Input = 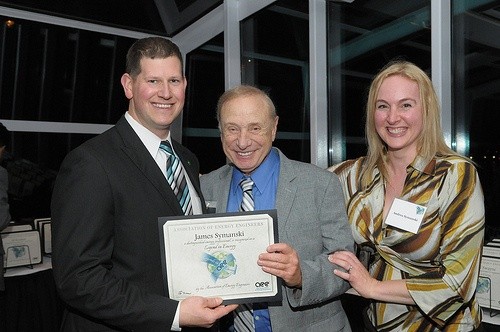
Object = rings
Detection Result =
[347,266,352,271]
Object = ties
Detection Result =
[233,178,255,332]
[159,140,194,216]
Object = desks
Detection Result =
[0,254,64,332]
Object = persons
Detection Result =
[51,38,239,332]
[199,85,354,332]
[0,123,9,295]
[326,60,485,332]
